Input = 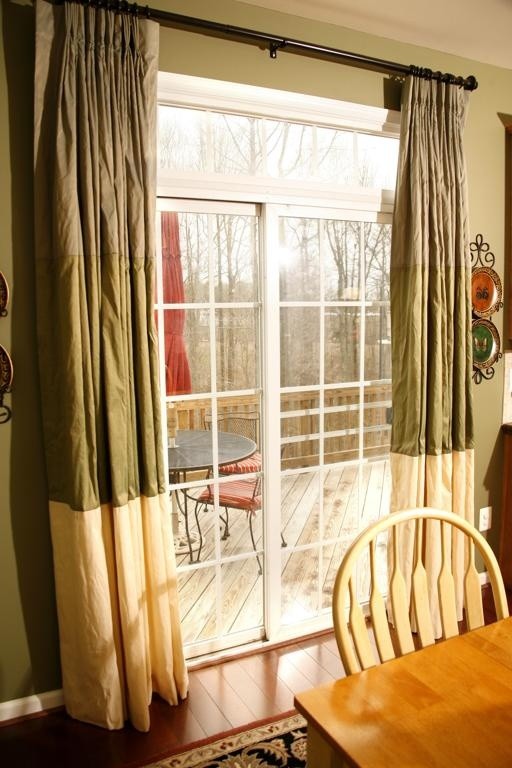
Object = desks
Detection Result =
[165,429,258,564]
[292,615,512,767]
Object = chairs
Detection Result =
[331,507,510,675]
[183,445,288,575]
[203,409,263,512]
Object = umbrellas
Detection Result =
[159,207,193,532]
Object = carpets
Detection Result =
[121,708,308,768]
